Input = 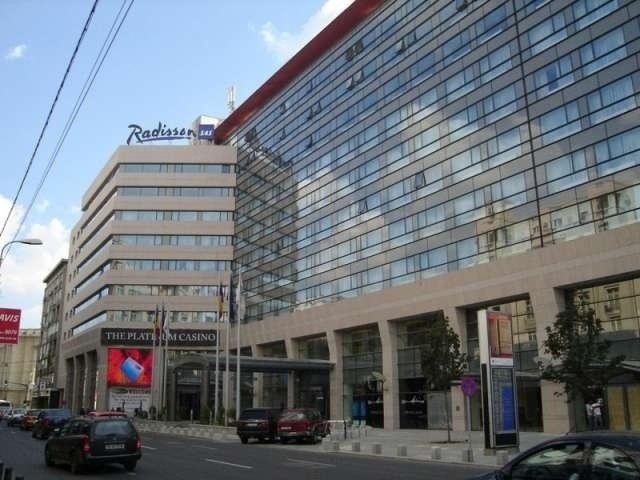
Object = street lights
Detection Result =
[4,380,29,409]
[0,237,44,267]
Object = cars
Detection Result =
[464,431,640,480]
[0,406,144,474]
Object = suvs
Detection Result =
[234,406,287,445]
[275,406,331,445]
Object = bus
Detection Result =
[0,400,13,417]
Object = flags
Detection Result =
[218,283,225,323]
[163,308,168,336]
[153,306,160,344]
[234,275,247,321]
